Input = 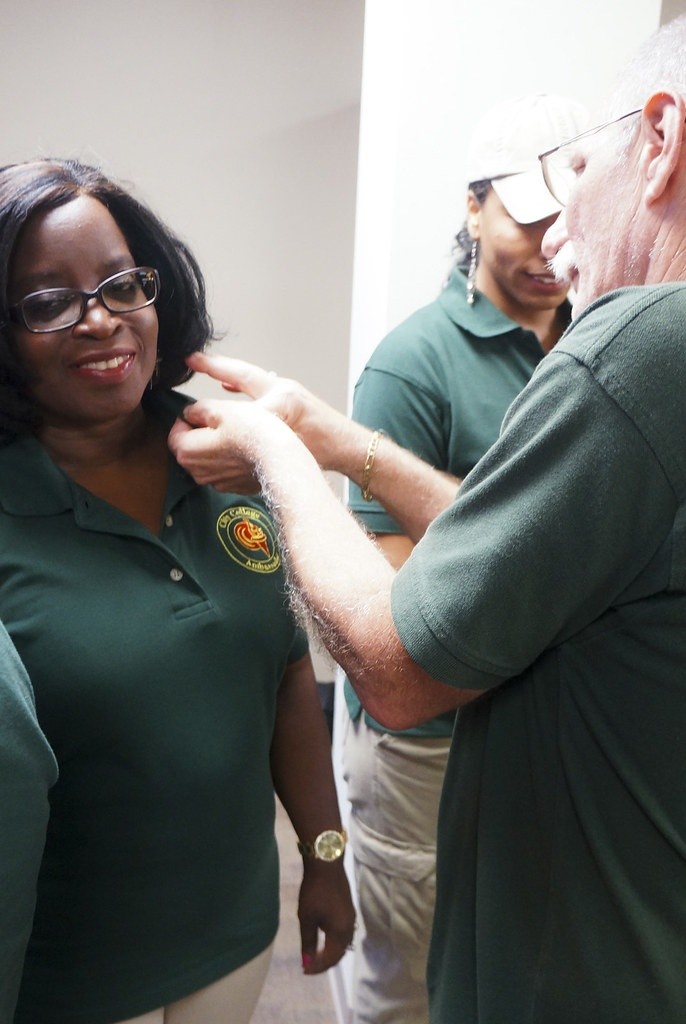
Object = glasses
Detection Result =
[16,267,159,333]
[542,107,642,208]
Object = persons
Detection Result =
[327,93,574,1024]
[0,157,363,1023]
[168,8,686,1024]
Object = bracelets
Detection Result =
[360,428,382,502]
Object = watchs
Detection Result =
[292,830,349,862]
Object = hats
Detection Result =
[469,143,564,224]
